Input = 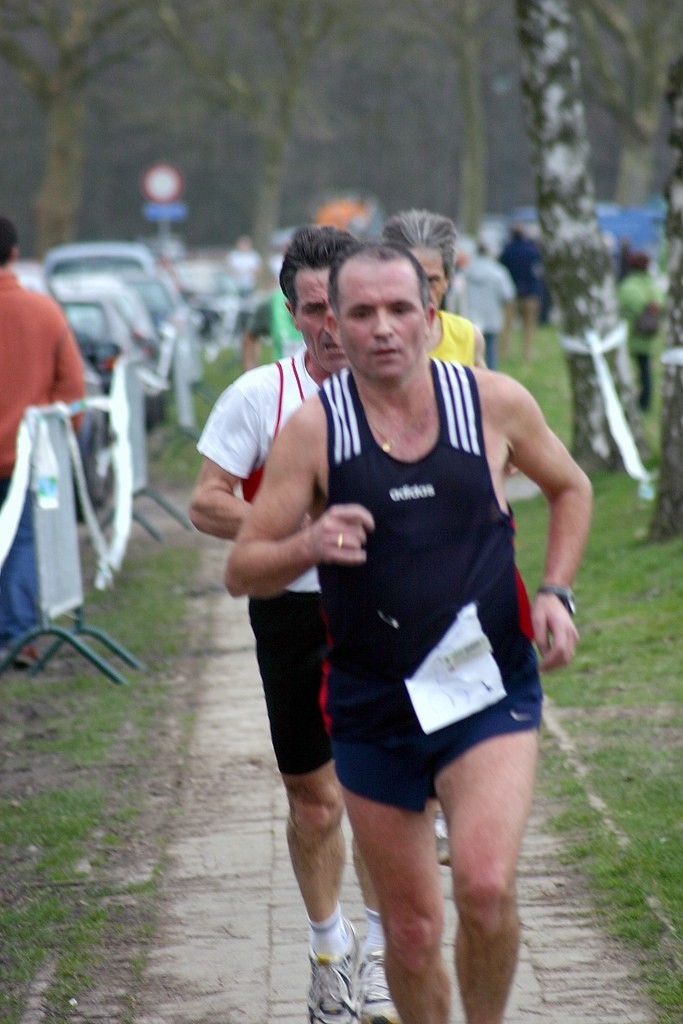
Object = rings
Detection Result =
[337,533,343,548]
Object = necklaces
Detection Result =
[363,400,418,452]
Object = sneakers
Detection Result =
[308,918,360,1024]
[357,949,400,1024]
[433,810,450,865]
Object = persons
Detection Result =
[500,224,547,357]
[222,239,592,1023]
[620,253,661,409]
[228,235,261,334]
[447,241,518,370]
[380,210,519,867]
[242,254,284,371]
[188,225,405,1024]
[0,218,84,667]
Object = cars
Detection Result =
[14,215,297,519]
[504,196,666,320]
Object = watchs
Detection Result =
[538,583,576,615]
